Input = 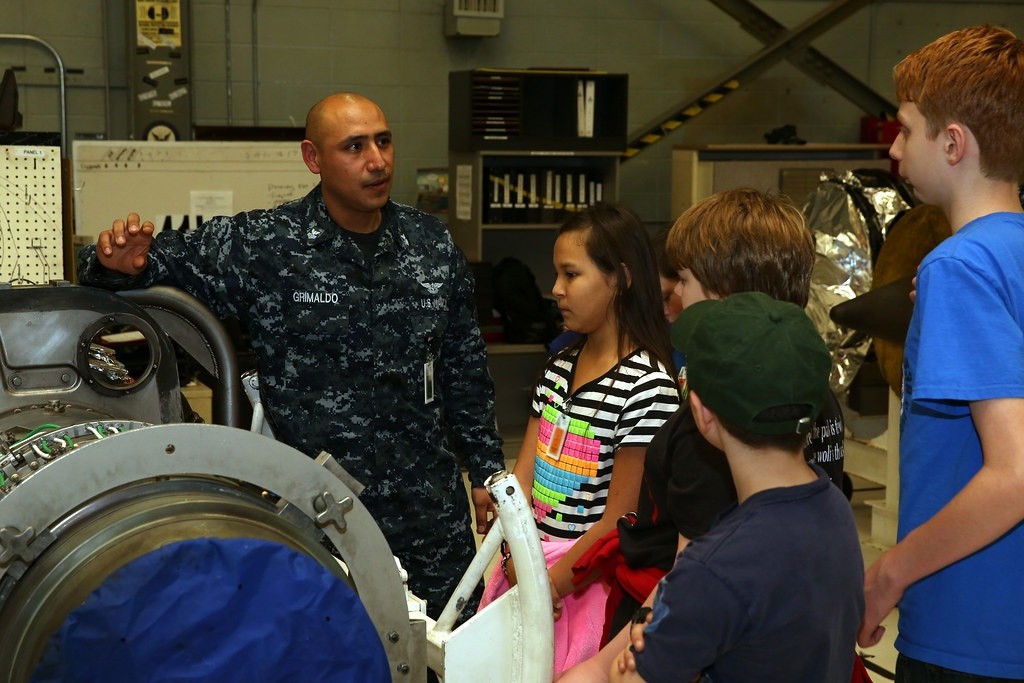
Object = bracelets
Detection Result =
[500,552,511,575]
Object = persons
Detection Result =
[607,290,865,683]
[475,200,679,679]
[77,91,505,683]
[553,186,845,683]
[857,24,1024,683]
